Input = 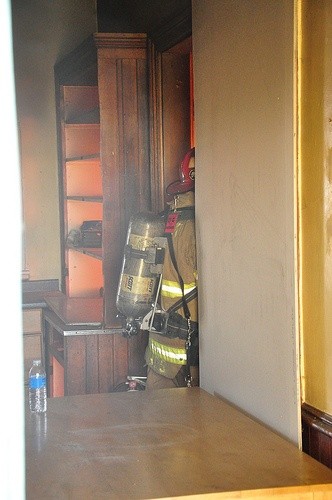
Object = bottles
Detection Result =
[28,359,47,413]
[115,210,166,337]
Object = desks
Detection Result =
[24,386,331,500]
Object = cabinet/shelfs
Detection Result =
[53,32,159,330]
[42,308,150,398]
[22,279,59,367]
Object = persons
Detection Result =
[145,146,201,388]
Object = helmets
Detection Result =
[166,146,195,196]
